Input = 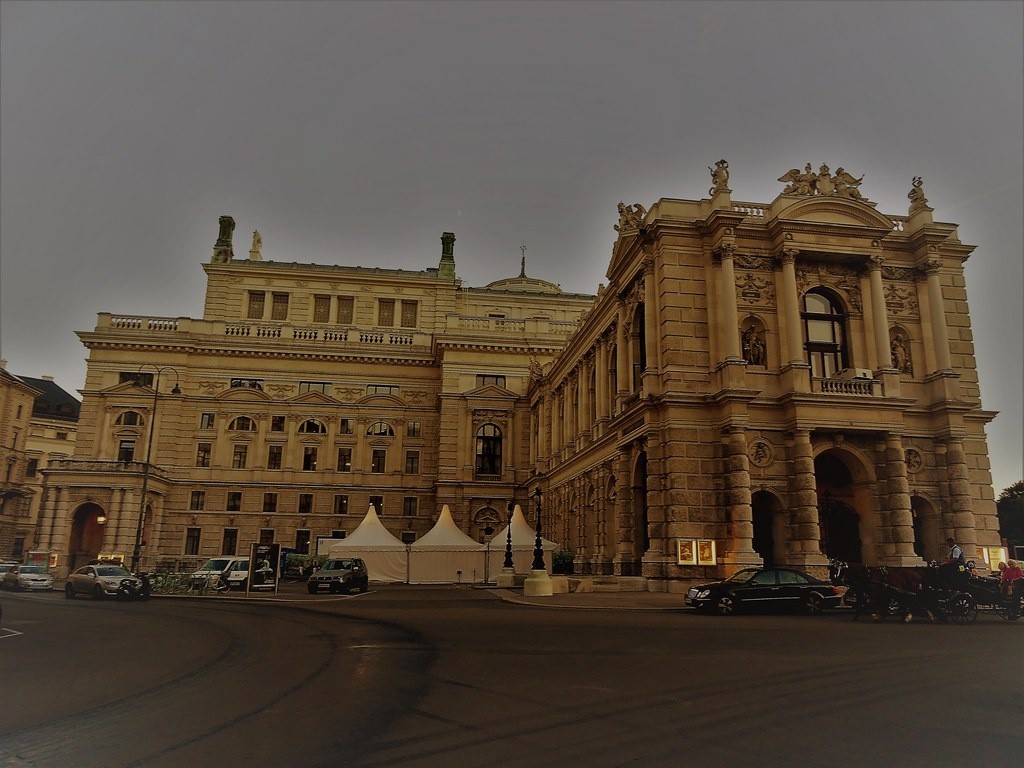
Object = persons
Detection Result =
[998,559,1023,600]
[617,202,643,232]
[908,176,928,214]
[890,333,908,373]
[780,162,817,195]
[743,324,764,365]
[708,159,729,194]
[831,167,865,198]
[251,229,262,251]
[940,538,964,568]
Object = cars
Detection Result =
[0,562,54,592]
[684,567,842,615]
[307,557,368,594]
[66,564,143,599]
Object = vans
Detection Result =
[191,557,250,589]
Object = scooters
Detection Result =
[117,570,151,600]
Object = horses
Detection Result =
[846,567,938,624]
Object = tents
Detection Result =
[328,504,557,585]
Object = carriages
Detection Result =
[827,563,1024,625]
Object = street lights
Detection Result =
[131,363,181,572]
[524,485,553,596]
[497,503,516,587]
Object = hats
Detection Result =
[944,538,955,542]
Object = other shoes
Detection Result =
[940,586,949,593]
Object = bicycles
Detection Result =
[148,564,231,595]
